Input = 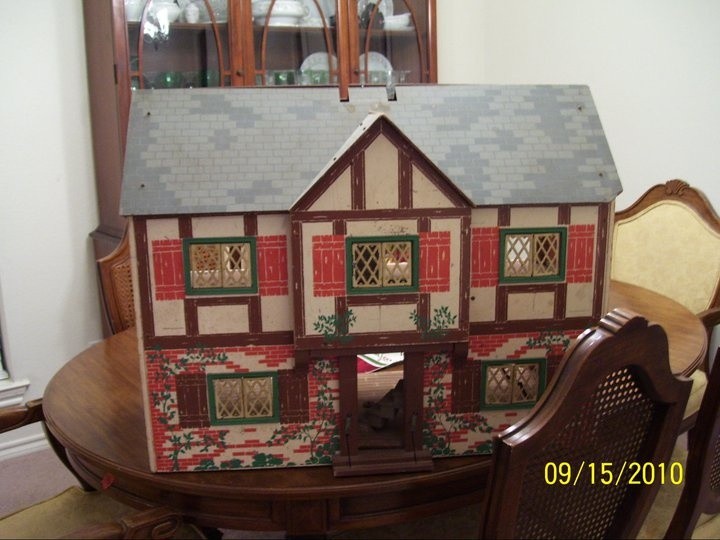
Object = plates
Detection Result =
[383,25,416,31]
[300,51,340,84]
[359,51,394,87]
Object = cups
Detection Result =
[131,67,413,90]
[184,3,199,22]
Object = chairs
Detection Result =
[667,346,720,540]
[93,220,135,339]
[606,179,719,316]
[1,398,209,540]
[362,309,693,540]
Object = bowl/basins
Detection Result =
[385,15,409,25]
[251,1,312,25]
[152,3,180,22]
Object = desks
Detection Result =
[43,281,707,540]
